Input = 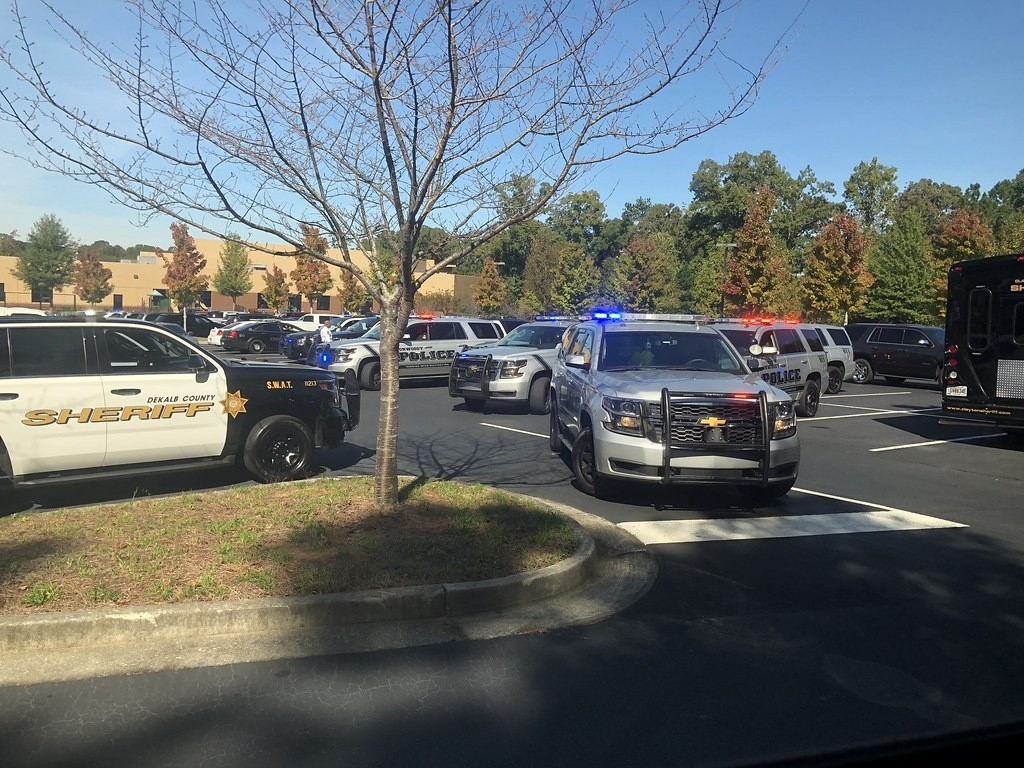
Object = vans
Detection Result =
[939,253,1024,436]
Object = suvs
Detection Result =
[304,313,506,390]
[0,311,364,516]
[447,314,585,415]
[279,315,381,360]
[702,317,830,419]
[545,310,800,504]
[842,323,945,386]
[807,323,856,394]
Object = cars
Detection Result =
[220,321,306,355]
[99,306,343,347]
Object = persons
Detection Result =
[233,316,239,322]
[321,321,332,343]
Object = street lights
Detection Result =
[715,243,739,318]
